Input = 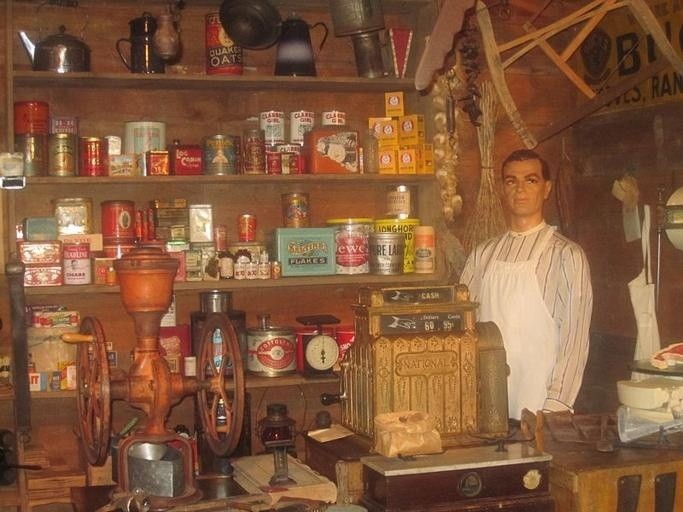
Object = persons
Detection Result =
[456,144,593,428]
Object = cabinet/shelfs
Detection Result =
[11,61,445,511]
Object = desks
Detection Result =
[513,412,682,512]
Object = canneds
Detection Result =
[200,290,231,316]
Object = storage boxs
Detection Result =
[304,126,360,175]
[264,227,336,277]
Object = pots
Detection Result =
[218,1,281,50]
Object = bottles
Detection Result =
[274,12,331,77]
[113,12,169,74]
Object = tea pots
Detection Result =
[17,25,94,72]
